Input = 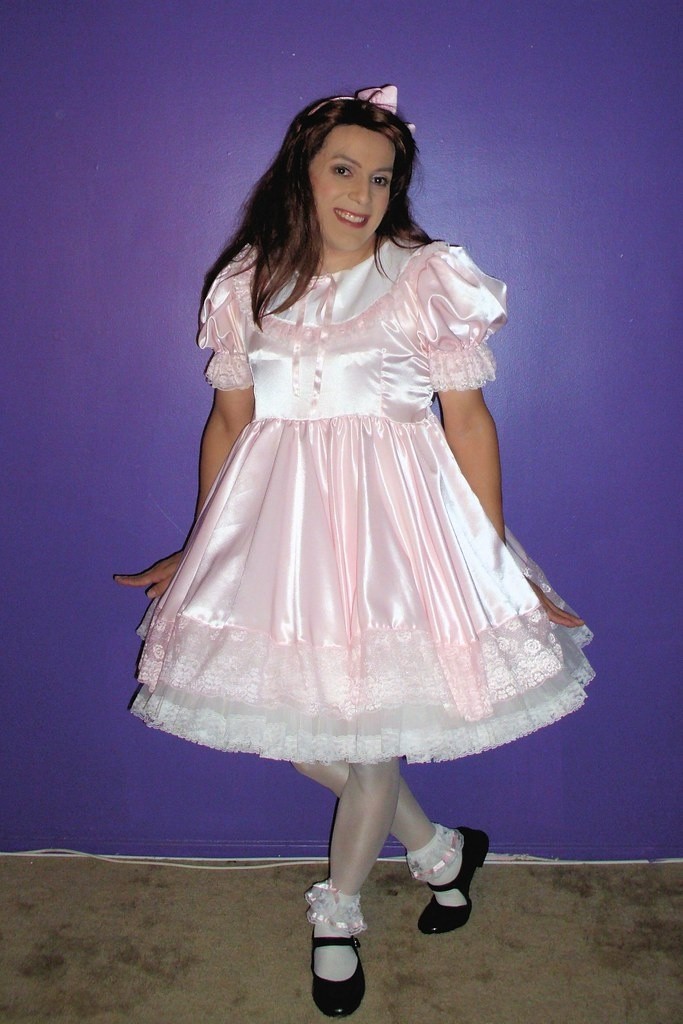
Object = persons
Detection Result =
[108,84,599,1019]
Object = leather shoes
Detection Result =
[310,920,365,1018]
[417,826,490,934]
